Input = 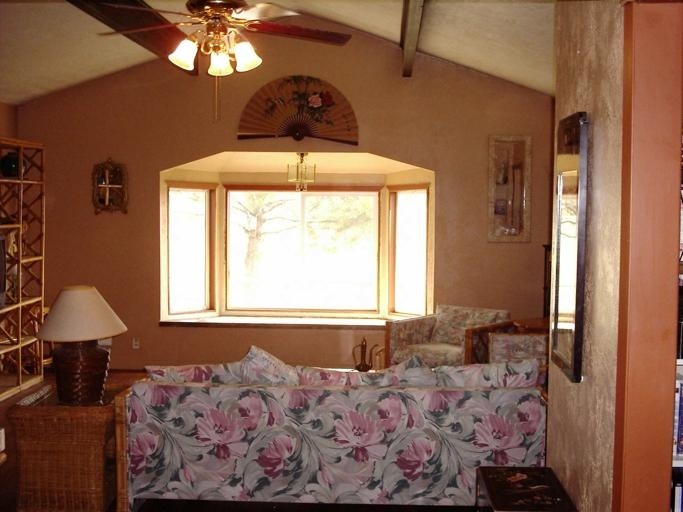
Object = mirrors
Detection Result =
[550,108,586,385]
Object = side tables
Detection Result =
[6,382,130,512]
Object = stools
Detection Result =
[474,465,579,511]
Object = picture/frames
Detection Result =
[92,156,127,215]
[487,135,533,244]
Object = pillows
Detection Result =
[432,358,537,385]
[294,366,435,385]
[242,346,301,385]
[143,360,240,382]
[380,356,422,372]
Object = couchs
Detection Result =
[126,381,546,508]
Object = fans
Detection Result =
[96,1,352,47]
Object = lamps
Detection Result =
[34,285,128,406]
[167,21,263,77]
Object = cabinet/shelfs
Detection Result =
[1,137,44,402]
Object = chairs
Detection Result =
[385,302,549,365]
